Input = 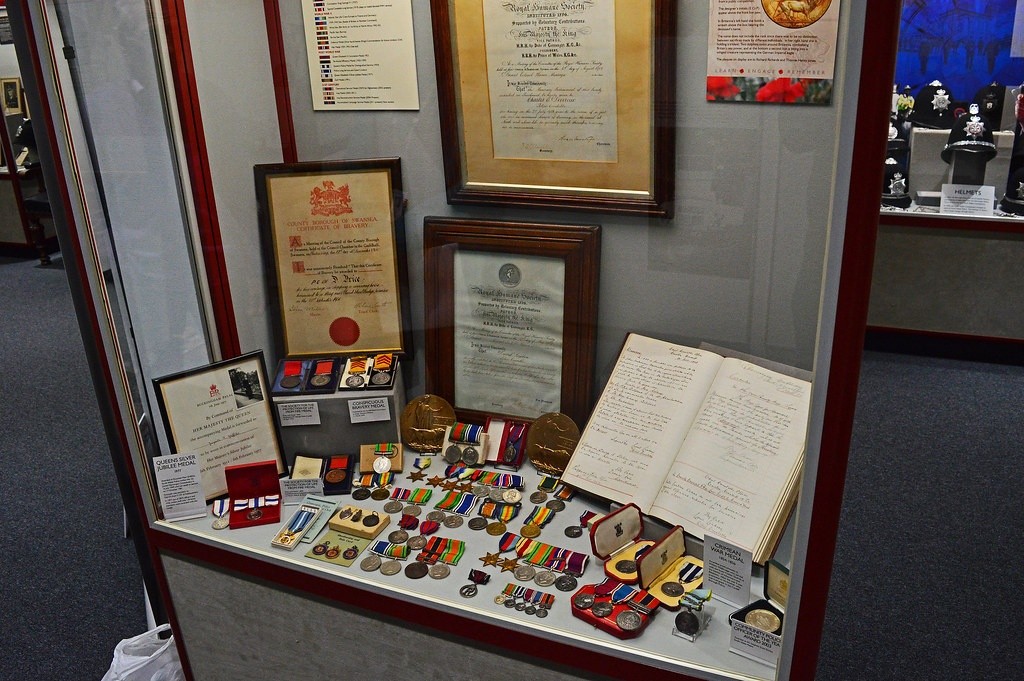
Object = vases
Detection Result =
[896,108,911,123]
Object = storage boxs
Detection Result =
[270,354,409,462]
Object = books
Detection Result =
[561,333,813,568]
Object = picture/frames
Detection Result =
[0,77,23,113]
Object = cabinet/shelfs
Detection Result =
[0,0,1024,681]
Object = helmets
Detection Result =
[939,101,1000,162]
[906,78,958,128]
[974,81,1014,135]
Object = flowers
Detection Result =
[897,93,915,110]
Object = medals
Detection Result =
[212,353,780,644]
